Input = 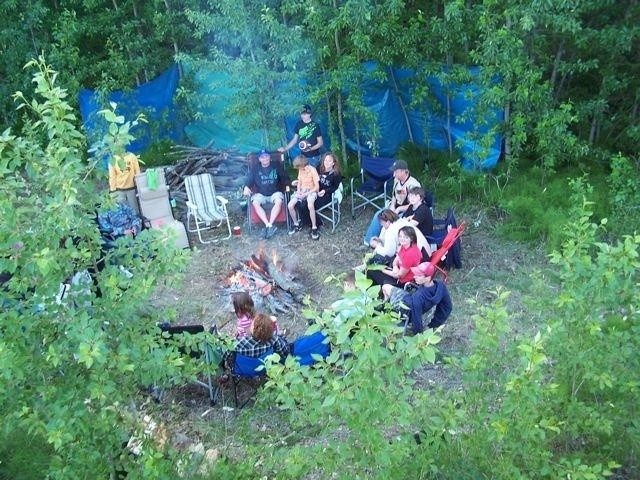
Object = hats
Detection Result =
[387,159,408,171]
[411,261,435,278]
[257,148,269,157]
[302,105,311,114]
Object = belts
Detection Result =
[116,187,137,192]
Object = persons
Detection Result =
[229,291,257,337]
[352,161,422,254]
[366,225,423,287]
[369,207,432,260]
[106,140,142,217]
[221,314,290,372]
[400,186,434,244]
[380,261,454,336]
[242,149,290,238]
[276,103,324,169]
[286,155,322,240]
[295,151,342,236]
[97,200,154,270]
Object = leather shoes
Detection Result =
[216,372,230,385]
[244,377,261,389]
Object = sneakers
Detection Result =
[288,220,324,240]
[120,269,133,278]
[265,225,277,238]
[257,226,267,241]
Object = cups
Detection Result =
[234,226,241,235]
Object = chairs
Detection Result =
[156,324,331,411]
[402,189,465,336]
[133,150,397,259]
